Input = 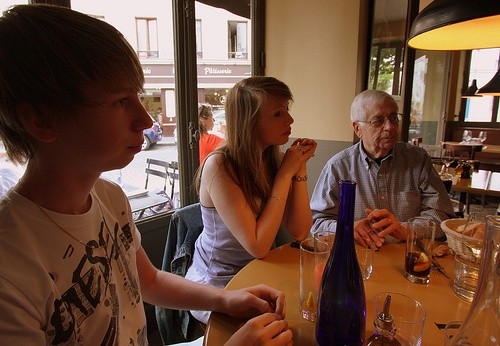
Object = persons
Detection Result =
[0,4,294,346]
[184,74,317,323]
[156,106,162,124]
[310,89,454,252]
[197,101,227,166]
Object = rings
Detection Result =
[301,148,305,154]
[388,217,392,223]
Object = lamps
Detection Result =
[407,0,500,51]
[461,79,482,98]
[474,68,500,96]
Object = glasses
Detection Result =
[357,113,404,128]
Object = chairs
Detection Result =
[443,144,482,161]
[136,157,179,219]
[155,203,206,345]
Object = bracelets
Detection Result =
[291,174,308,182]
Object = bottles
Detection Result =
[364,294,401,346]
[454,161,471,179]
[447,215,500,345]
[316,180,364,346]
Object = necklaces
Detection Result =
[38,191,110,249]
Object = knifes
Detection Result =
[417,239,451,280]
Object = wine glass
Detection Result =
[441,149,455,173]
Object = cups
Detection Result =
[445,320,497,346]
[365,291,425,346]
[438,173,453,195]
[299,230,377,322]
[441,218,487,303]
[463,129,487,146]
[404,217,436,282]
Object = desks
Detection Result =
[202,234,500,346]
[443,141,500,162]
[434,164,500,196]
[129,192,169,219]
